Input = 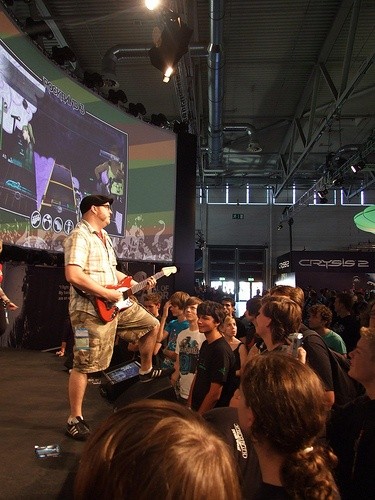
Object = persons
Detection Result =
[245,297,267,352]
[236,351,341,500]
[21,123,35,170]
[75,400,241,500]
[326,327,375,500]
[217,315,247,390]
[57,283,236,371]
[95,147,124,234]
[229,296,307,406]
[309,304,347,360]
[188,301,235,413]
[0,238,18,337]
[254,276,375,359]
[64,194,167,439]
[156,292,190,370]
[127,293,163,360]
[171,297,207,404]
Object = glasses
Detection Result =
[94,205,113,211]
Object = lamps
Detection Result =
[351,161,365,173]
[148,21,194,73]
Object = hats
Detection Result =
[80,194,114,214]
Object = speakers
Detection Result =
[113,375,179,417]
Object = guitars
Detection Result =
[95,265,177,324]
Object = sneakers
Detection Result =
[64,416,92,441]
[138,367,175,384]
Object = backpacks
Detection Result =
[303,330,365,410]
